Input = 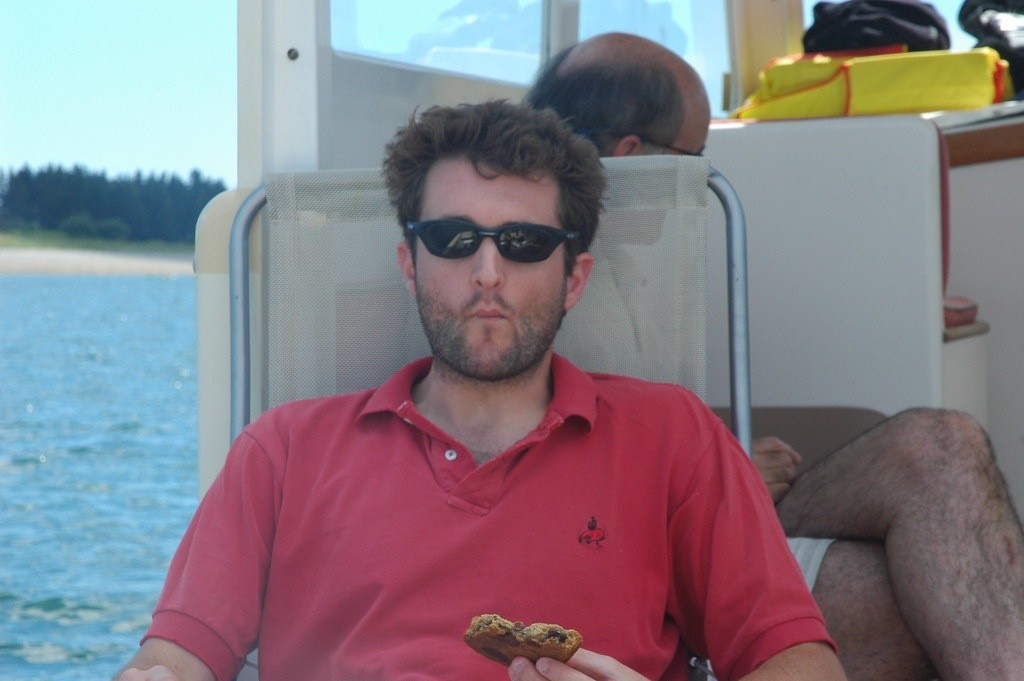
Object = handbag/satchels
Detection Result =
[800,1,950,52]
[731,41,1008,121]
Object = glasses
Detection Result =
[405,219,581,264]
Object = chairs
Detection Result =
[229,153,755,462]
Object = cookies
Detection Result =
[463,614,584,666]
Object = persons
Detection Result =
[104,102,845,681]
[519,0,1024,679]
